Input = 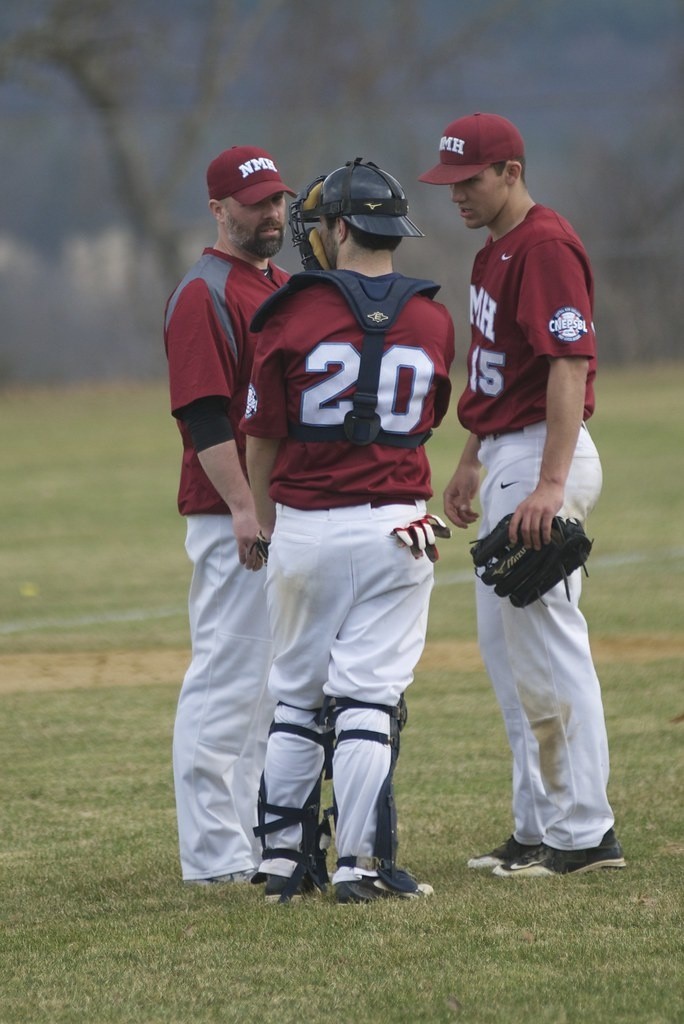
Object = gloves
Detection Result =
[392,514,452,564]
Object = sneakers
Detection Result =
[194,866,258,890]
[254,874,317,905]
[468,824,629,880]
[336,865,433,901]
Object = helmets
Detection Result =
[322,166,422,244]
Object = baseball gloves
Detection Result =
[470,513,594,611]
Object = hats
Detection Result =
[419,112,524,183]
[206,146,297,208]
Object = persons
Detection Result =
[161,146,323,881]
[412,112,627,875]
[230,160,459,907]
